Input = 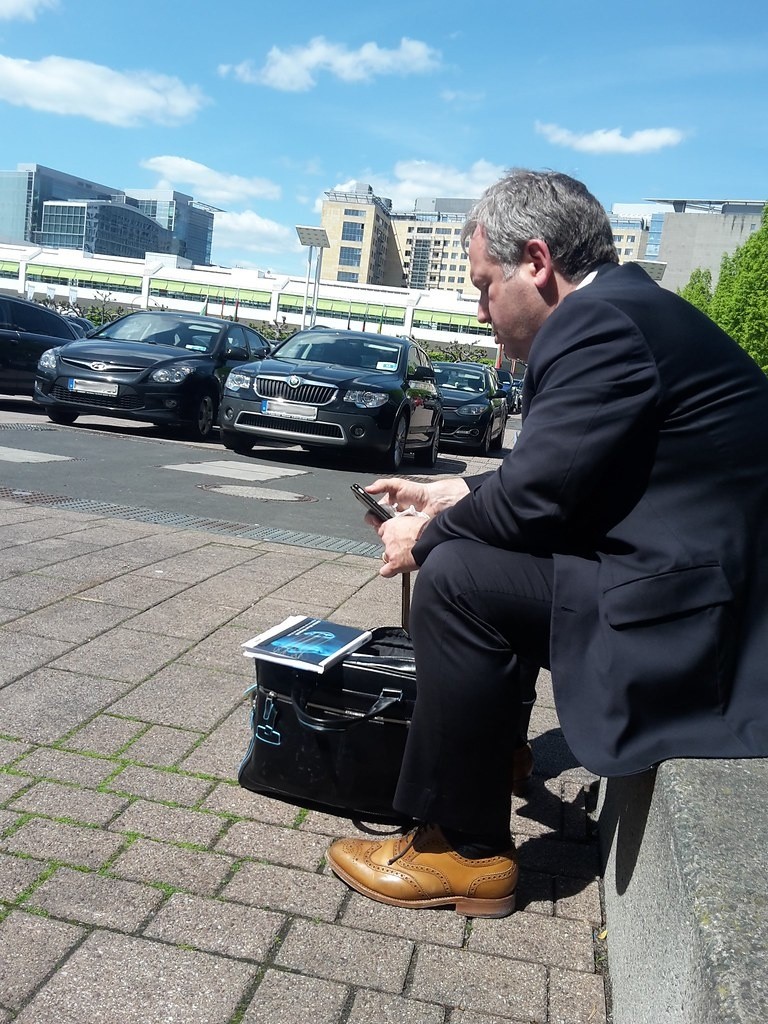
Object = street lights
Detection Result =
[296,225,331,327]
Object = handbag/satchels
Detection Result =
[237,626,423,820]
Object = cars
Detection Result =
[215,323,445,471]
[0,294,83,412]
[61,315,97,339]
[32,310,272,444]
[429,358,523,456]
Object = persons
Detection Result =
[326,167,767,920]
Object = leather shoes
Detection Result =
[324,821,519,920]
[512,744,534,794]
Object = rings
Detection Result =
[382,552,388,563]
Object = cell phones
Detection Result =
[351,483,393,521]
